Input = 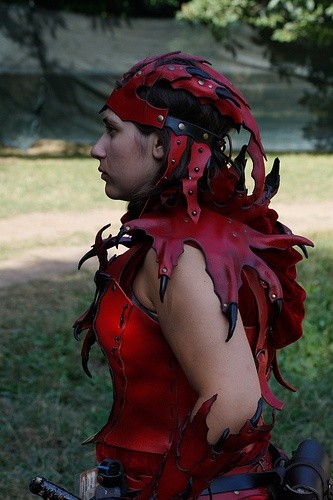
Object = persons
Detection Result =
[70,50,315,500]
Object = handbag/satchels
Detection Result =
[278,440,332,500]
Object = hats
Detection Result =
[99,51,281,224]
